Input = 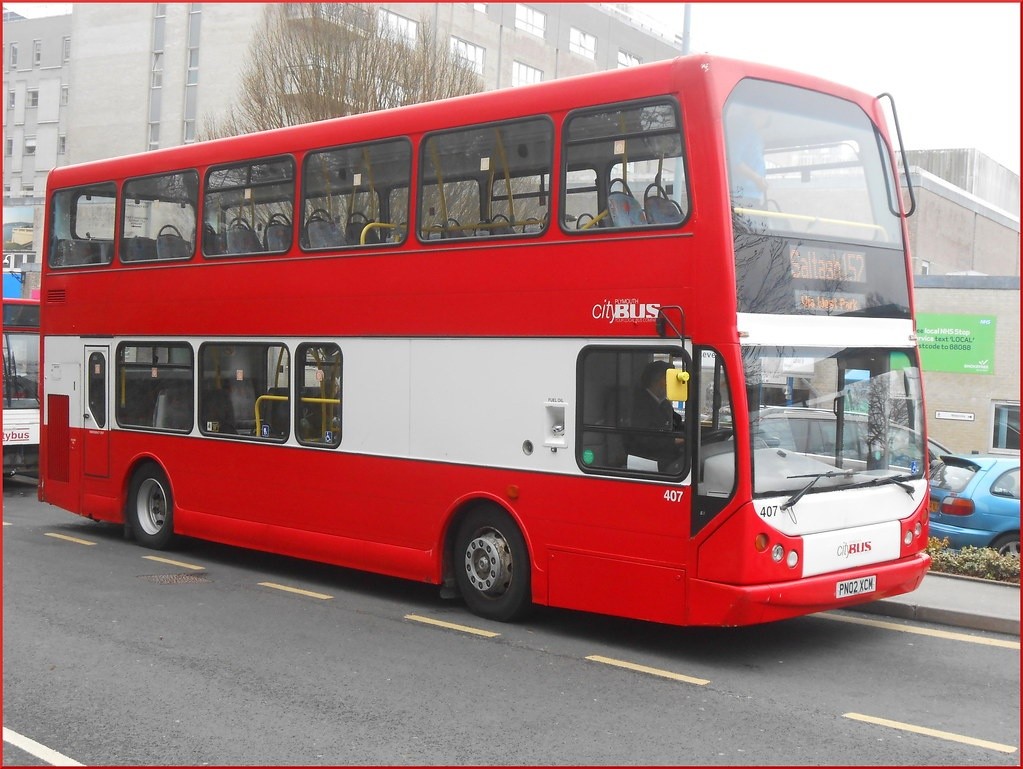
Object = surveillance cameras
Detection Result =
[3,260,9,264]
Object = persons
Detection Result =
[614,361,703,471]
[309,378,340,445]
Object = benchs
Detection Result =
[154,383,196,431]
[224,379,263,429]
[55,234,157,266]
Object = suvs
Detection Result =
[704,402,953,474]
[928,451,1021,559]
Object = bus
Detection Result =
[2,297,40,475]
[37,56,933,626]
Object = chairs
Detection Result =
[263,212,293,253]
[576,212,597,229]
[190,223,220,257]
[604,387,634,466]
[523,216,543,234]
[644,183,680,223]
[224,218,262,254]
[426,218,466,241]
[346,211,379,247]
[606,178,647,226]
[474,221,490,234]
[305,209,346,250]
[489,214,516,235]
[157,225,187,259]
